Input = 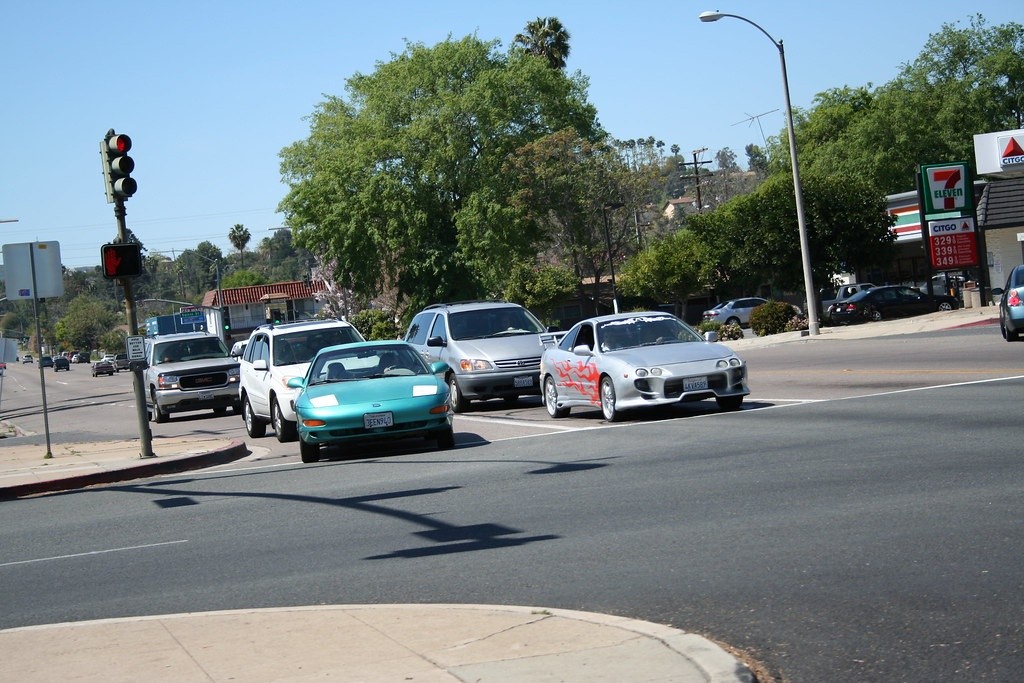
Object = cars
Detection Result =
[703,297,801,329]
[991,265,1024,341]
[287,340,454,463]
[23,354,33,364]
[539,312,750,422]
[803,275,965,328]
[51,351,90,363]
[37,356,69,372]
[91,361,113,377]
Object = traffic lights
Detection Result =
[104,133,138,198]
[222,306,231,340]
[271,308,282,324]
[101,242,143,279]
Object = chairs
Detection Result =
[328,361,346,380]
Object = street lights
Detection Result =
[699,10,820,335]
[152,249,222,310]
[602,203,624,314]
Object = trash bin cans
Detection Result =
[962,288,972,309]
[970,289,981,308]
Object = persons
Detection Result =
[277,334,346,362]
[597,325,672,352]
[159,342,217,363]
[378,353,403,376]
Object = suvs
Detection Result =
[231,317,380,443]
[143,331,240,424]
[101,353,130,372]
[402,299,558,413]
